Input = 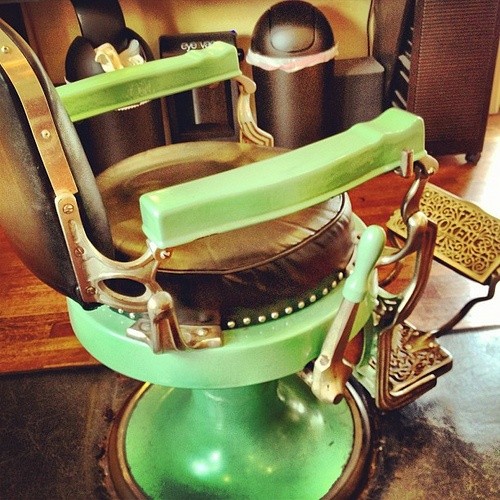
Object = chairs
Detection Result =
[0,21,500,500]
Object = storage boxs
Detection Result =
[333,56,385,132]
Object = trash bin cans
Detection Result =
[158,28,245,141]
[245,0,338,152]
[62,0,168,177]
